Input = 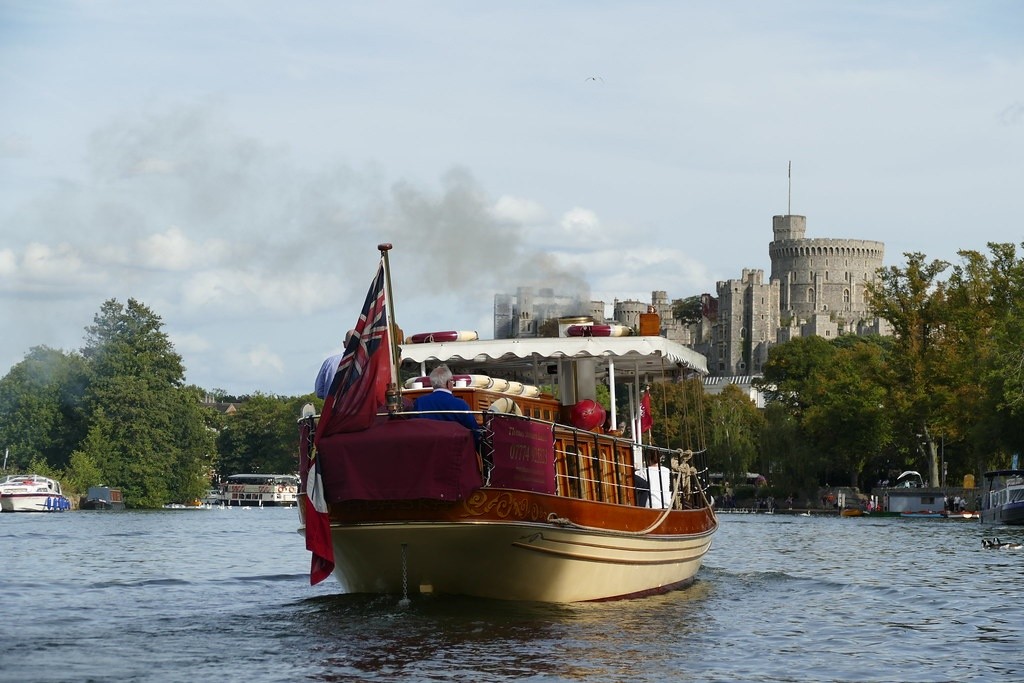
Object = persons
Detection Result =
[414,364,479,430]
[822,480,982,512]
[635,444,672,509]
[616,414,632,439]
[314,329,356,401]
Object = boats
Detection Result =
[841,508,863,517]
[0,473,70,512]
[217,473,298,507]
[202,490,221,503]
[981,537,1021,549]
[183,497,201,506]
[288,238,722,606]
[977,468,1024,526]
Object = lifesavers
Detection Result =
[404,374,540,388]
[405,330,478,344]
[558,322,635,337]
[481,397,522,477]
[289,486,295,493]
[278,486,284,492]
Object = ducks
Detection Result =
[981,537,1024,551]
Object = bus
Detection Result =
[709,473,768,496]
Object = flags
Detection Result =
[298,265,390,585]
[635,392,653,434]
